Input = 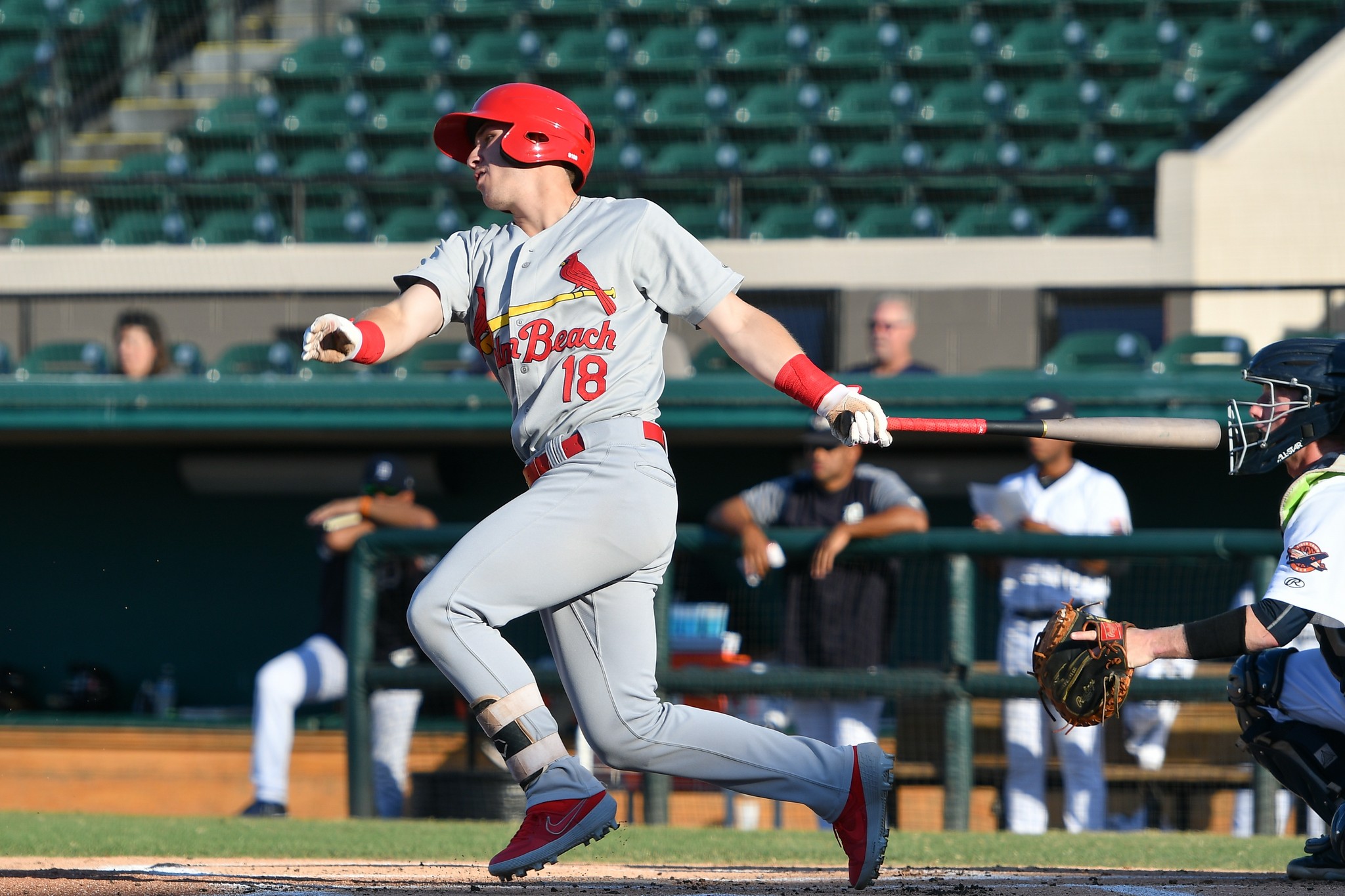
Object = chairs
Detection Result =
[1039,331,1152,373]
[1151,335,1250,373]
[690,336,748,374]
[17,338,484,375]
[0,0,1345,242]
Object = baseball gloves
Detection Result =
[1032,608,1137,727]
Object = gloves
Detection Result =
[301,313,362,361]
[817,384,892,448]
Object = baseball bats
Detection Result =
[840,410,1221,451]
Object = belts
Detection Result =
[1012,603,1085,621]
[522,421,665,489]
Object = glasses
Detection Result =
[868,321,893,329]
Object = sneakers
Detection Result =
[488,790,618,881]
[832,743,895,890]
[1287,853,1345,881]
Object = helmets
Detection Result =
[433,82,595,193]
[1226,337,1345,475]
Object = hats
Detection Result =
[364,460,418,494]
[1023,393,1074,420]
[799,413,841,446]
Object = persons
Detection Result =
[1069,336,1345,883]
[972,391,1132,834]
[1121,656,1203,769]
[714,408,930,828]
[113,308,171,378]
[239,454,443,817]
[301,82,895,890]
[841,298,940,376]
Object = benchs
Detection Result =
[874,663,1261,835]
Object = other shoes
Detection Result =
[243,801,285,818]
